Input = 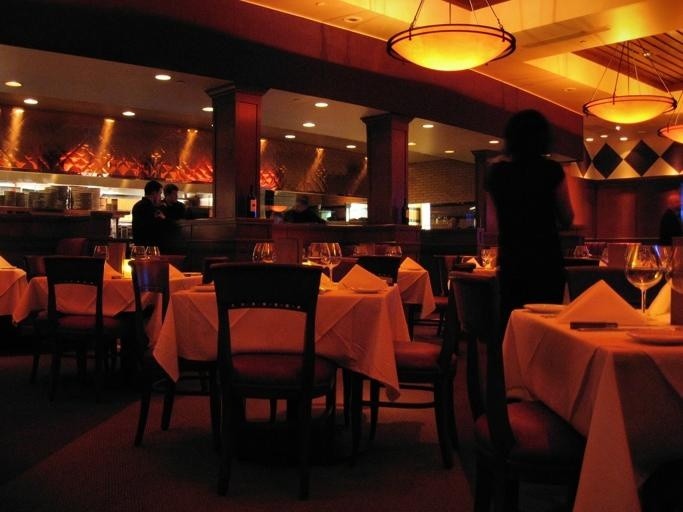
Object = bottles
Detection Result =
[106,197,119,211]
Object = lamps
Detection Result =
[582,39,678,125]
[386,0,517,72]
[656,90,683,145]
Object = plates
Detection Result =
[193,285,216,292]
[626,327,683,347]
[352,288,378,293]
[0,185,107,213]
[523,303,566,312]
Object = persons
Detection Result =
[658,189,682,247]
[160,184,185,245]
[479,109,574,340]
[284,197,326,223]
[131,180,165,248]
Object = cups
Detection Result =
[131,246,161,259]
[94,246,110,262]
[251,243,277,264]
[666,246,683,295]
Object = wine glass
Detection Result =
[481,249,496,271]
[309,243,343,283]
[624,242,665,322]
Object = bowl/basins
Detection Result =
[455,263,476,272]
